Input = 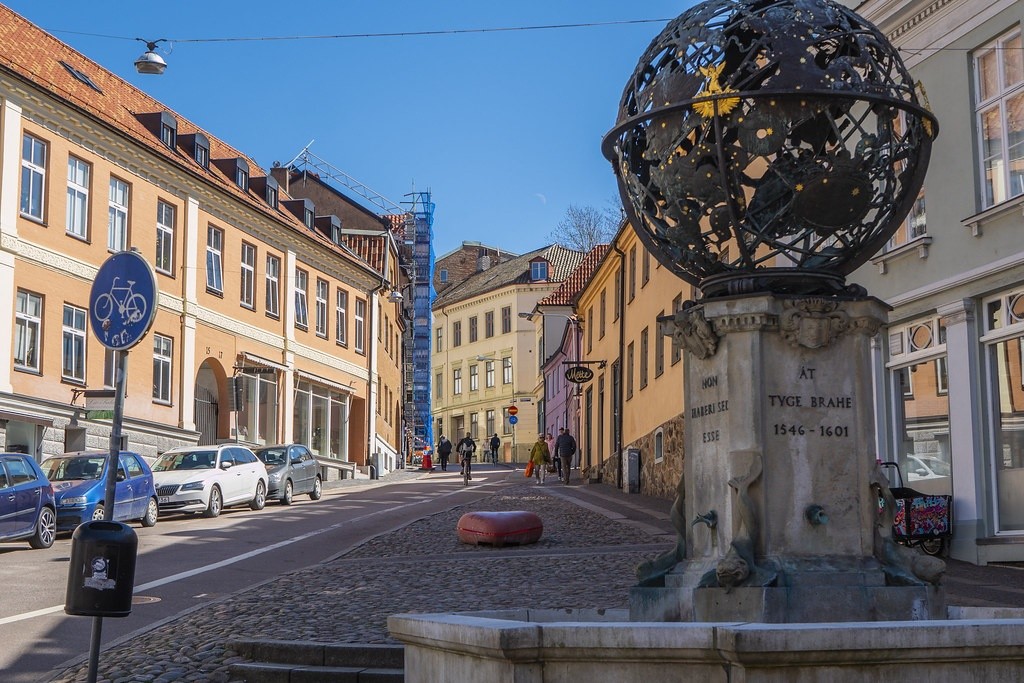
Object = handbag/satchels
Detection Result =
[525,461,534,477]
[552,456,561,469]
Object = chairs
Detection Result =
[63,460,99,479]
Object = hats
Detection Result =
[559,427,564,431]
[484,438,488,440]
[539,433,544,437]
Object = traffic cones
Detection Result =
[419,445,436,471]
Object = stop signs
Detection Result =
[508,406,518,415]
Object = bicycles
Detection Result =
[485,451,490,462]
[458,451,474,486]
[490,447,499,467]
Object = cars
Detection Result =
[907,453,951,482]
[39,450,159,531]
[254,443,322,505]
[149,443,268,517]
[0,452,57,548]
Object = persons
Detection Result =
[490,433,500,463]
[482,439,490,463]
[437,435,452,471]
[198,454,210,466]
[555,427,576,484]
[176,456,190,467]
[97,464,123,482]
[545,434,555,472]
[456,431,476,480]
[530,433,550,484]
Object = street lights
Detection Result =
[477,355,519,462]
[517,311,580,468]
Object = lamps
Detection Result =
[385,285,404,303]
[130,37,174,75]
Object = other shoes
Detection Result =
[562,478,564,481]
[536,480,540,484]
[468,475,472,480]
[460,470,464,475]
[558,477,561,480]
[566,481,569,485]
[541,482,545,484]
